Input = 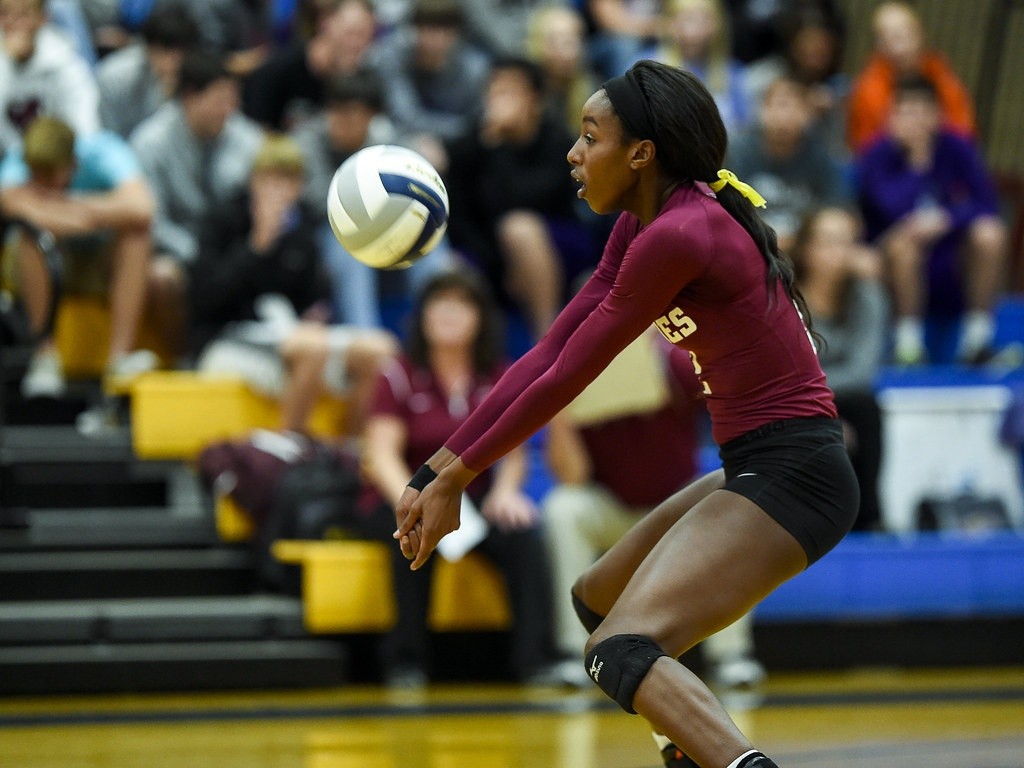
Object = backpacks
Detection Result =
[197,424,363,540]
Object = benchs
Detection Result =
[46,293,1024,625]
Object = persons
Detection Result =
[0,0,1024,696]
[395,58,860,768]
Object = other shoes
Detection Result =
[736,752,781,768]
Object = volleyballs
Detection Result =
[325,143,450,271]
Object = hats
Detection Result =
[251,132,312,175]
[173,56,231,95]
[22,117,75,164]
